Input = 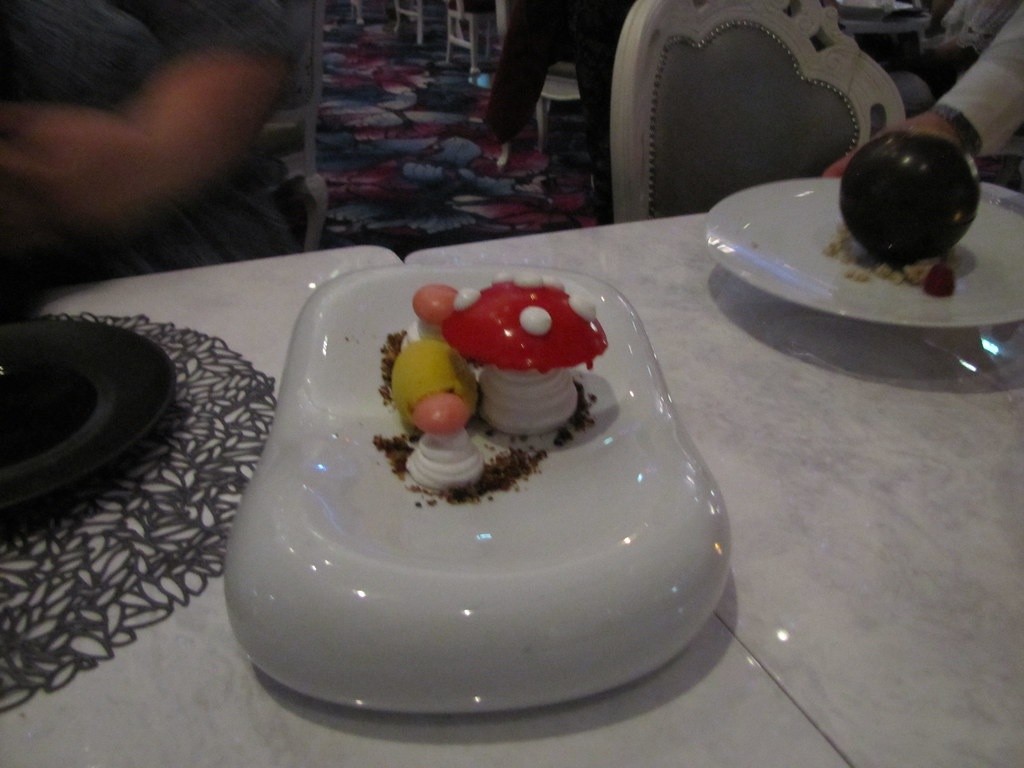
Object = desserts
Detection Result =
[377,270,611,507]
[834,127,983,300]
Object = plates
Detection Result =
[703,176,1024,328]
[222,260,733,718]
[1,320,177,511]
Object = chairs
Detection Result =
[608,1,908,226]
[259,1,335,252]
[348,0,587,154]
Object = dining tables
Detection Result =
[0,178,1021,765]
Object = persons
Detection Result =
[0,0,312,308]
[823,0,1024,179]
[483,0,631,225]
